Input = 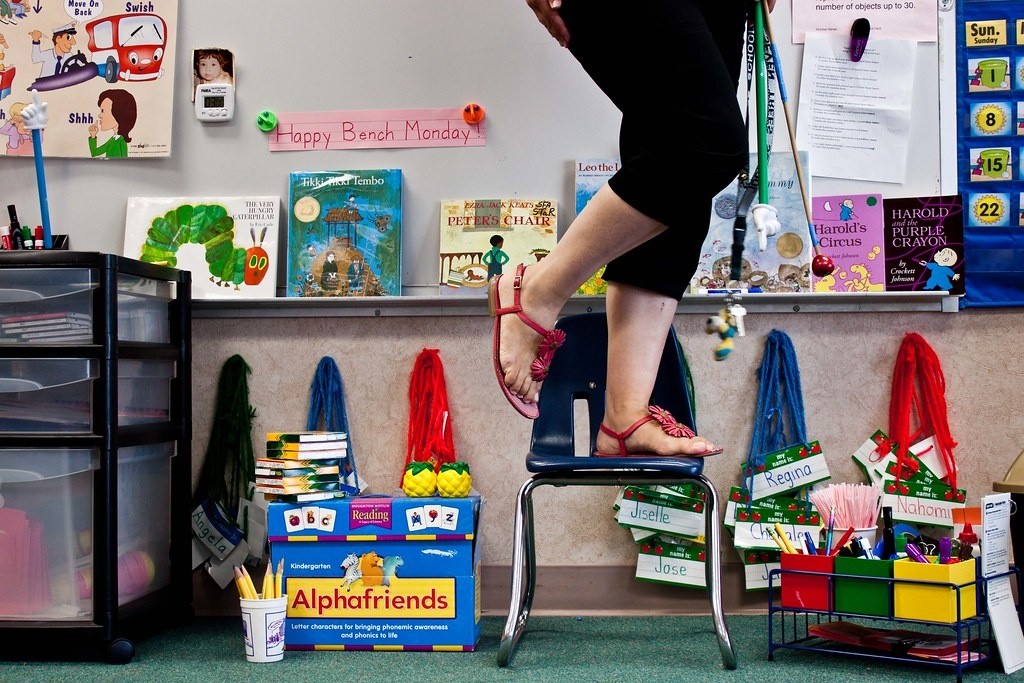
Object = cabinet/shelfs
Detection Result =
[769,563,1024,683]
[0,250,198,662]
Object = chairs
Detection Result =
[495,311,738,671]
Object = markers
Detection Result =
[906,537,952,564]
[7,203,44,250]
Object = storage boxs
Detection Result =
[891,556,979,624]
[834,556,910,617]
[267,493,478,541]
[780,548,841,610]
[270,541,484,651]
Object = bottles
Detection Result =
[958,521,980,558]
[896,534,907,557]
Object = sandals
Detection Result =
[489,263,565,419]
[594,405,724,458]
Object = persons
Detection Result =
[193,49,233,96]
[487,1,777,459]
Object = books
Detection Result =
[880,196,967,296]
[252,431,351,505]
[437,197,559,296]
[689,150,814,295]
[0,312,92,347]
[573,156,624,216]
[286,167,403,299]
[811,194,886,294]
[121,196,280,301]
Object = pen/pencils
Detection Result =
[232,558,285,598]
[766,507,854,555]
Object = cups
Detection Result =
[824,526,878,553]
[240,594,288,662]
